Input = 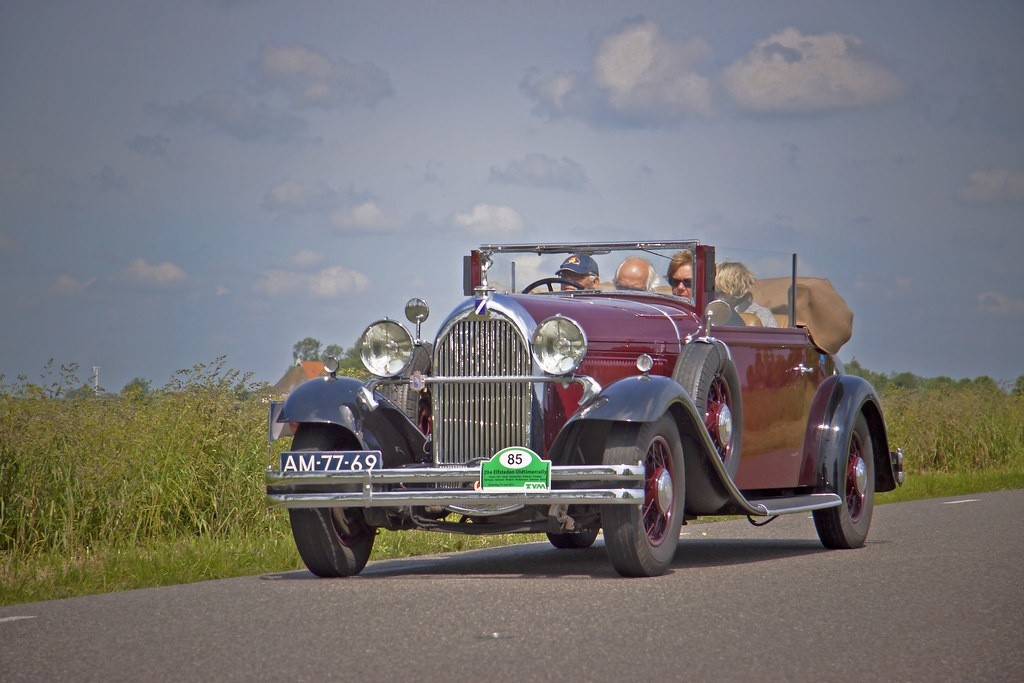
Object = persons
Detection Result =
[714,256,777,328]
[555,255,600,292]
[664,251,694,298]
[612,257,661,292]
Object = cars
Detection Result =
[264,236,908,579]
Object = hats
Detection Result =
[556,254,599,276]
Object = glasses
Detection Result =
[669,277,692,288]
[616,283,647,291]
[559,272,595,280]
